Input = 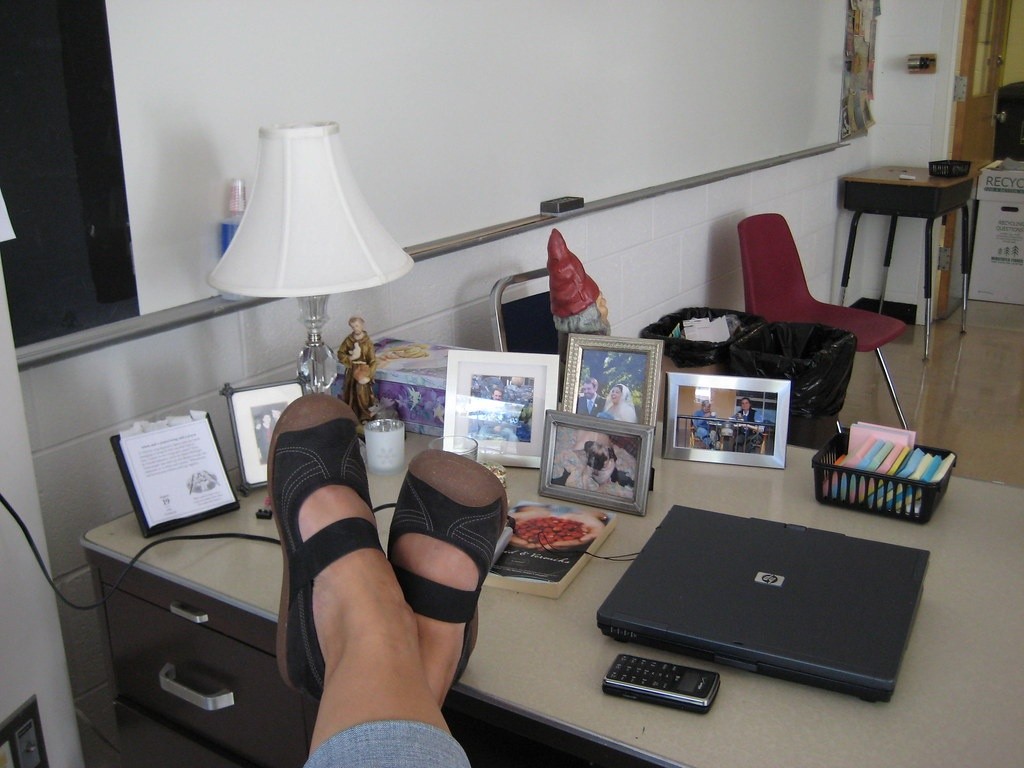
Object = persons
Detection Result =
[576,376,637,423]
[266,390,509,768]
[469,372,532,442]
[338,318,378,421]
[255,408,282,461]
[692,397,765,451]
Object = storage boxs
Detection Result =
[332,332,480,439]
[970,158,1024,304]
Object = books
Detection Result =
[483,500,618,599]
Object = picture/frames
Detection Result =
[538,410,656,516]
[221,374,311,496]
[662,371,792,470]
[443,347,560,467]
[562,332,664,429]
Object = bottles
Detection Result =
[222,180,250,300]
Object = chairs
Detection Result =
[738,213,909,435]
[487,268,559,356]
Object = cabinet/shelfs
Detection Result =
[81,445,1024,768]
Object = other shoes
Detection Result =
[266,392,387,696]
[386,447,508,685]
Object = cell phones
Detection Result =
[602,654,721,715]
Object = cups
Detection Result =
[429,436,478,462]
[364,419,405,476]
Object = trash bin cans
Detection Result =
[639,305,767,426]
[728,322,858,452]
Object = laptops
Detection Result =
[597,505,930,704]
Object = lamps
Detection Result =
[209,122,413,393]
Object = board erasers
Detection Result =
[539,195,585,216]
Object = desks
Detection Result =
[842,164,981,358]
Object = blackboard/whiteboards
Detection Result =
[0,0,851,371]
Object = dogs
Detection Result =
[561,441,617,483]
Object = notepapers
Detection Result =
[824,421,955,512]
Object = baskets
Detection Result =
[929,160,971,178]
[812,433,956,523]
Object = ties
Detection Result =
[588,400,593,414]
